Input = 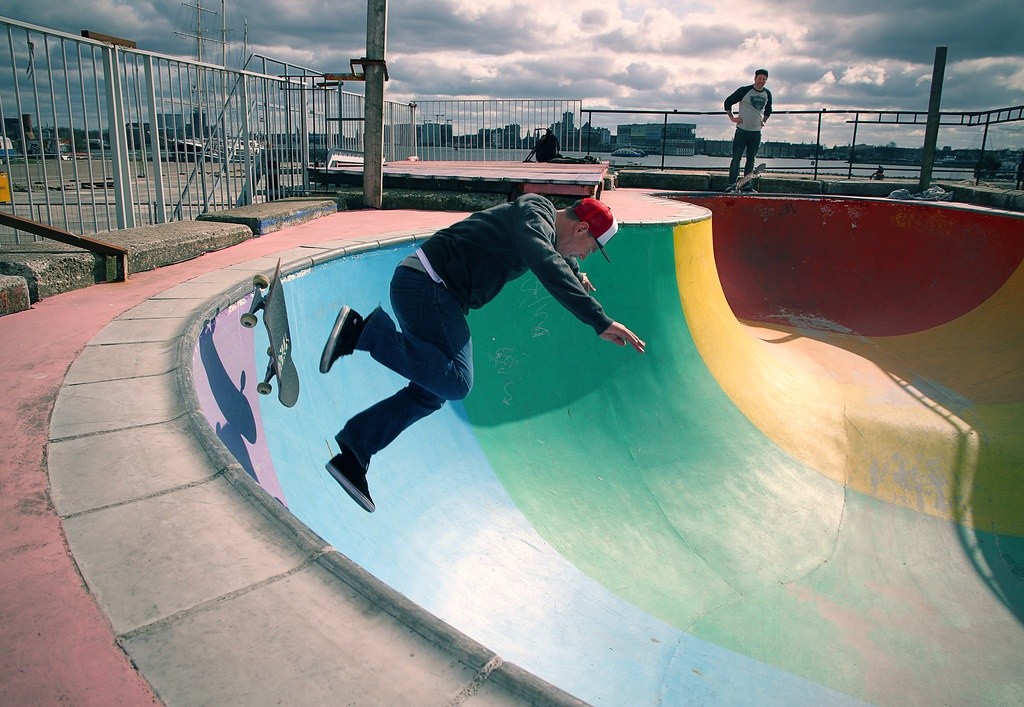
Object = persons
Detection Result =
[320,193,648,513]
[724,68,772,194]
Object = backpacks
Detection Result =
[523,127,563,163]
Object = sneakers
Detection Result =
[325,454,376,513]
[318,306,367,374]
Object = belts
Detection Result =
[398,257,430,274]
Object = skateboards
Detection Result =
[727,163,766,194]
[239,256,300,408]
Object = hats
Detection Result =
[572,198,619,264]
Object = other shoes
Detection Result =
[742,189,759,194]
[726,187,740,193]
